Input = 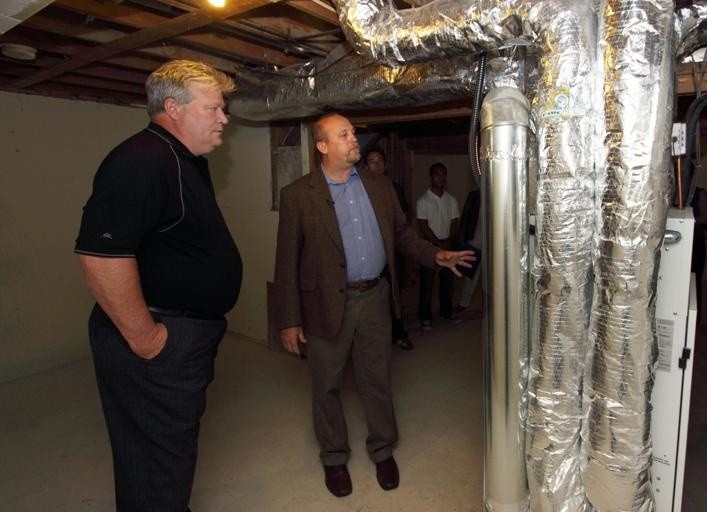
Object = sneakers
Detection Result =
[420,321,433,331]
[438,315,463,323]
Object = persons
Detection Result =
[415,161,466,333]
[452,189,481,313]
[272,113,479,498]
[72,60,244,512]
[360,146,413,352]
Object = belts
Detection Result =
[148,305,225,321]
[345,274,383,293]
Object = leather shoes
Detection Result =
[323,463,352,497]
[376,456,400,491]
[396,338,412,350]
